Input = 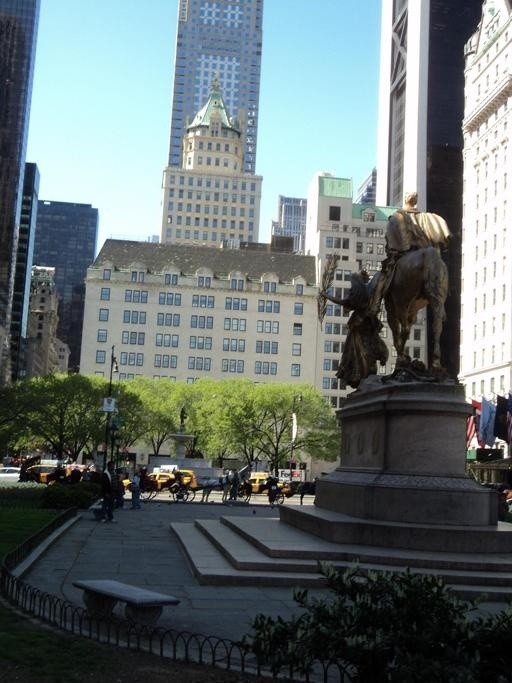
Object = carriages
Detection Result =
[129,464,316,506]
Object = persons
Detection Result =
[243,478,253,504]
[221,475,233,501]
[228,472,240,499]
[111,466,128,510]
[266,475,277,508]
[128,467,142,510]
[299,481,306,504]
[48,461,103,484]
[200,476,212,502]
[99,459,118,524]
[316,270,390,388]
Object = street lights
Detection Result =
[290,391,302,468]
[102,344,118,471]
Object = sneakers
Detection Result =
[97,517,117,524]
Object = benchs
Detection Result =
[72,579,181,626]
[88,499,108,521]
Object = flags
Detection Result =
[465,391,512,452]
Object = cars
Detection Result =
[121,470,291,495]
[0,465,72,484]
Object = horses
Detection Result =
[384,246,449,380]
[200,472,242,504]
